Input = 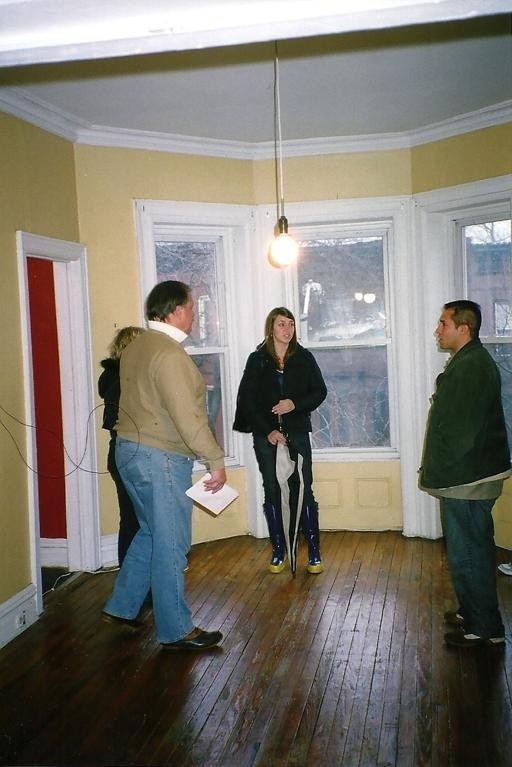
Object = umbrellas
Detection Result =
[273,411,307,579]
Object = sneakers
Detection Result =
[442,626,508,648]
[443,609,470,626]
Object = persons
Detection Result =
[97,326,151,603]
[495,559,512,580]
[231,306,331,578]
[414,299,511,649]
[101,279,227,652]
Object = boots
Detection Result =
[300,501,324,574]
[261,500,291,574]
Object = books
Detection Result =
[184,470,239,517]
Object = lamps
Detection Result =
[354,285,376,303]
[260,40,302,265]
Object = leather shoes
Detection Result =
[161,624,225,652]
[100,610,143,632]
